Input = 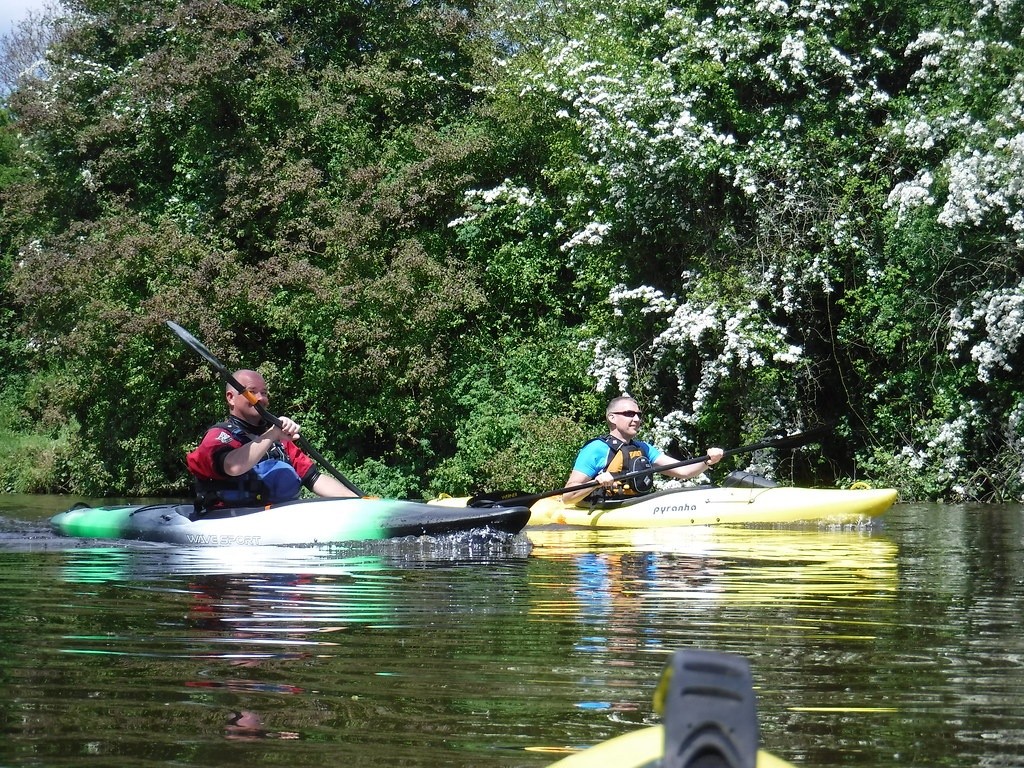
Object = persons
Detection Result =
[562,397,723,505]
[185,369,360,510]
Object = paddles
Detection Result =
[165,317,371,498]
[466,416,846,510]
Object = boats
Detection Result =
[427,471,901,529]
[51,498,532,547]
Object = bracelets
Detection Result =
[704,461,708,465]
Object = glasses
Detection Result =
[611,410,642,420]
